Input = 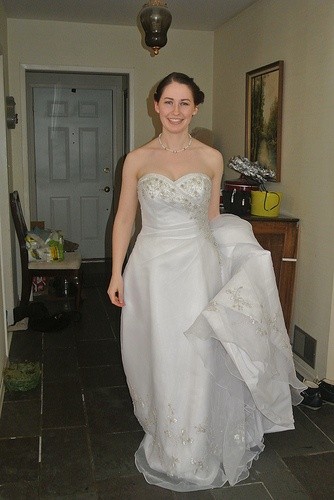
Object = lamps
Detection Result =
[140,0,173,55]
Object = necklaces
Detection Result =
[158,132,191,153]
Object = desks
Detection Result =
[220,208,300,343]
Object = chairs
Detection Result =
[9,190,82,326]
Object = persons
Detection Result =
[107,72,307,491]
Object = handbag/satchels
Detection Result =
[222,188,250,217]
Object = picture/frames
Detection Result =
[245,60,284,183]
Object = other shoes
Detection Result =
[297,379,323,411]
[312,377,334,406]
